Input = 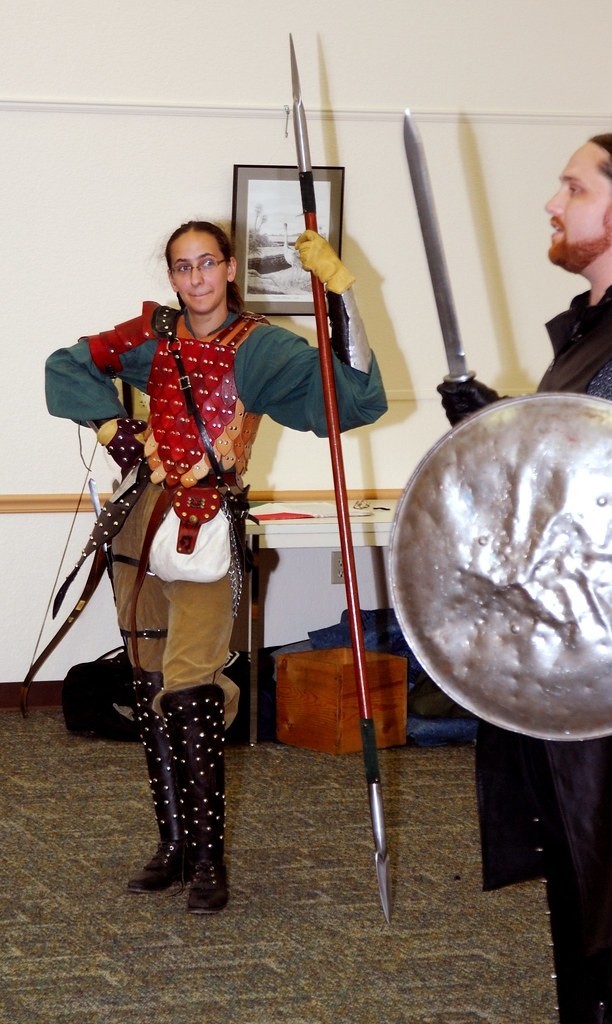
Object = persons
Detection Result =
[42,221,388,916]
[433,129,611,1024]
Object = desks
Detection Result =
[245,498,397,745]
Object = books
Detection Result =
[244,500,375,521]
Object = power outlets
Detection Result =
[329,550,344,584]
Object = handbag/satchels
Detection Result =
[62,645,279,747]
[228,485,260,573]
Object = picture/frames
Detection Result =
[229,163,345,315]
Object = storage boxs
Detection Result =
[276,647,406,755]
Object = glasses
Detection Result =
[170,256,226,277]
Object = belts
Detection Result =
[130,470,244,675]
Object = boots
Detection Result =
[126,669,192,897]
[160,683,230,913]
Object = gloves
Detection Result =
[295,229,356,295]
[437,379,508,428]
[97,416,149,471]
